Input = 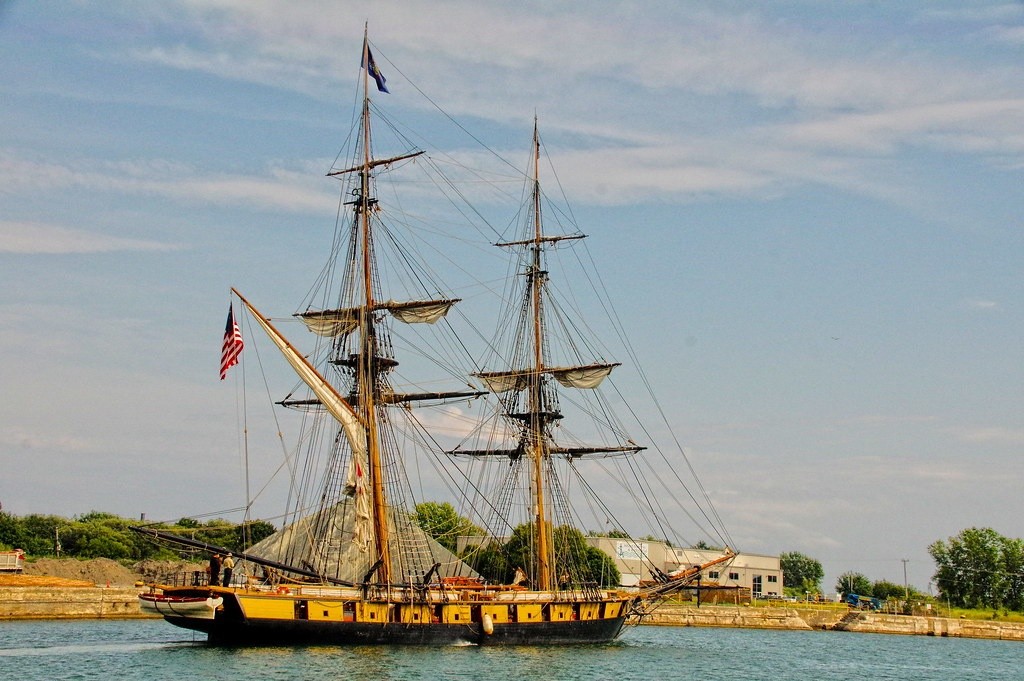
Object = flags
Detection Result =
[219,301,244,381]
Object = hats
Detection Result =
[226,553,233,556]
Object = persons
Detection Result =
[222,552,235,587]
[209,552,221,586]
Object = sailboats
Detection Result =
[124,19,740,648]
